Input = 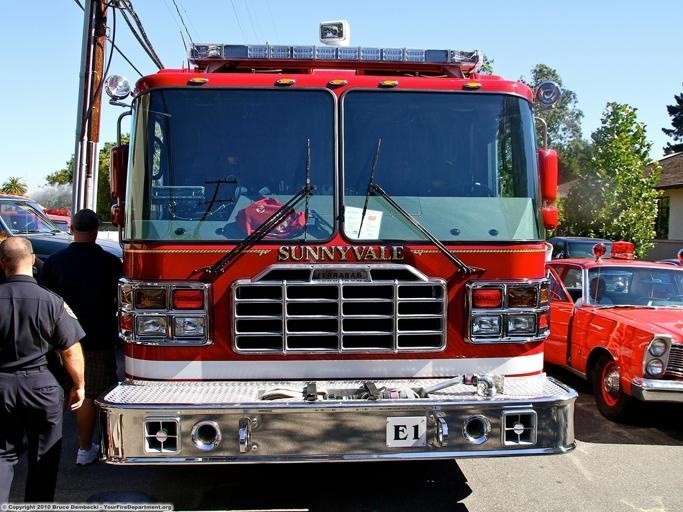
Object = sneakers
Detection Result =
[77,443,100,466]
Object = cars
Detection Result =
[0,194,124,278]
[543,235,683,427]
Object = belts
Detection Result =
[22,364,48,371]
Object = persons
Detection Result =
[0,209,123,502]
[574,276,614,307]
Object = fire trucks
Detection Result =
[92,20,580,469]
